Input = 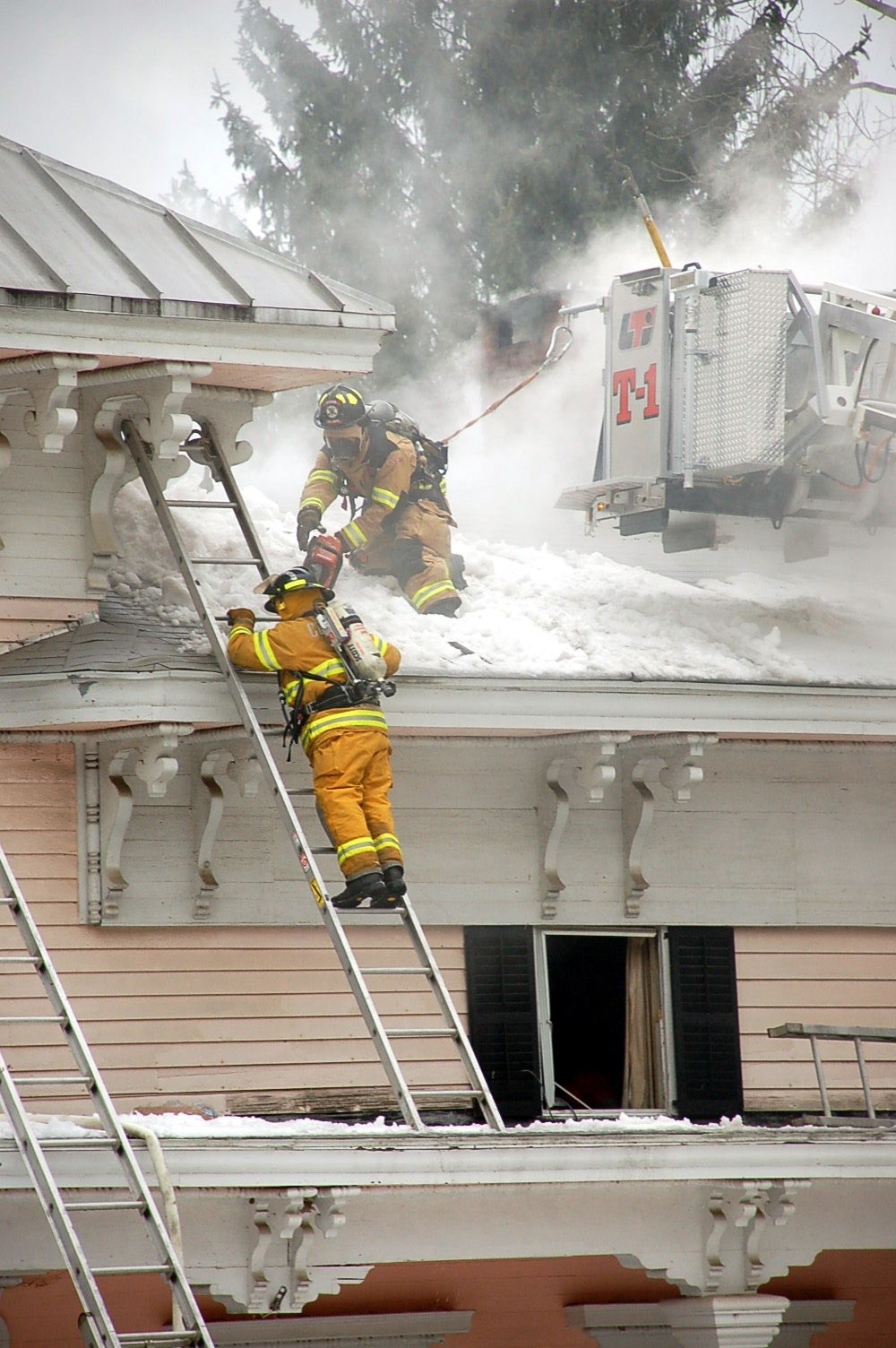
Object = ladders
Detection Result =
[768,1022,895,1126]
[0,855,212,1347]
[120,413,507,1133]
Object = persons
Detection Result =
[227,566,407,909]
[297,383,468,619]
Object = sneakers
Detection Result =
[330,873,386,907]
[370,867,407,908]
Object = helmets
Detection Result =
[263,566,336,614]
[314,385,367,430]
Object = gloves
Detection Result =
[335,532,351,554]
[297,511,326,551]
[227,608,256,625]
